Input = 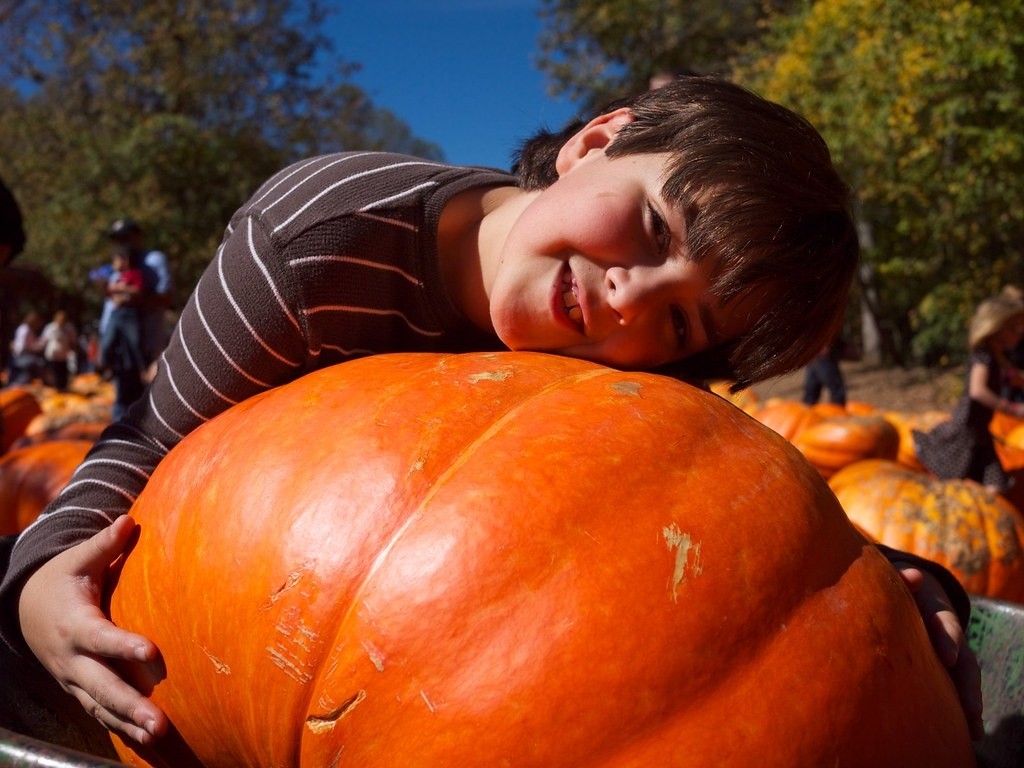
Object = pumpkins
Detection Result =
[101,352,974,768]
[710,381,1024,607]
[0,371,115,537]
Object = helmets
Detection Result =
[107,218,140,239]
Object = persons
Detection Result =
[805,325,850,405]
[0,177,182,424]
[910,296,1024,497]
[0,70,984,768]
